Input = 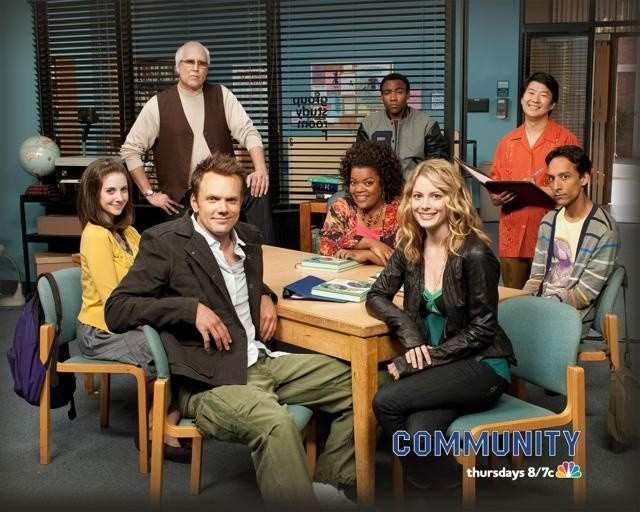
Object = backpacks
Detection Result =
[6,273,77,421]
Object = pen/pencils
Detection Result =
[530,167,544,177]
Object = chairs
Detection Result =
[391,295,587,512]
[37,266,154,474]
[142,324,318,511]
[577,264,626,372]
[299,201,328,254]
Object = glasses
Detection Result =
[180,59,209,69]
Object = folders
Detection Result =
[453,154,557,209]
[283,275,348,303]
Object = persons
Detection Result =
[77,159,192,463]
[522,145,619,340]
[365,159,518,512]
[319,141,406,266]
[356,73,448,182]
[490,72,582,397]
[121,41,274,245]
[104,151,395,511]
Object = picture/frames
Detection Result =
[310,60,395,130]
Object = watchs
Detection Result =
[143,189,154,197]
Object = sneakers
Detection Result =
[312,482,359,512]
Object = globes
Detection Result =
[18,135,60,194]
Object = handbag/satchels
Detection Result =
[595,264,640,454]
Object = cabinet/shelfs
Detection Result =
[20,189,165,301]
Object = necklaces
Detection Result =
[361,203,386,228]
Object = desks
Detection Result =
[259,244,535,511]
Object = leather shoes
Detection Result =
[148,440,188,464]
[134,430,139,450]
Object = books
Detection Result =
[306,177,344,184]
[295,263,366,272]
[302,255,360,270]
[312,182,343,191]
[312,278,372,303]
[315,193,334,199]
